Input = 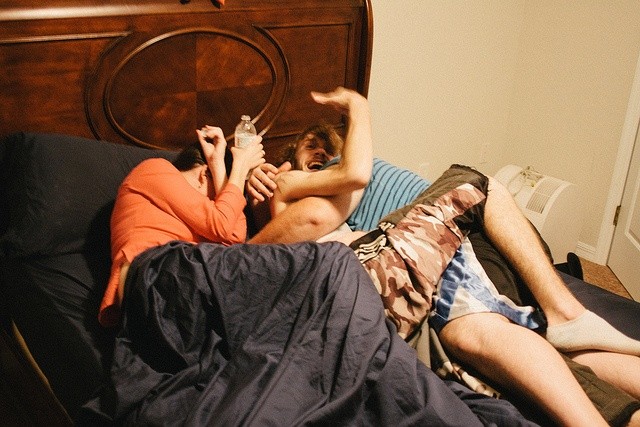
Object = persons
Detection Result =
[245,153,640,427]
[96,125,265,327]
[269,86,640,427]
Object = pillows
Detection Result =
[0,131,180,261]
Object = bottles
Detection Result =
[234,115,257,180]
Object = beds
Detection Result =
[1,0,640,427]
